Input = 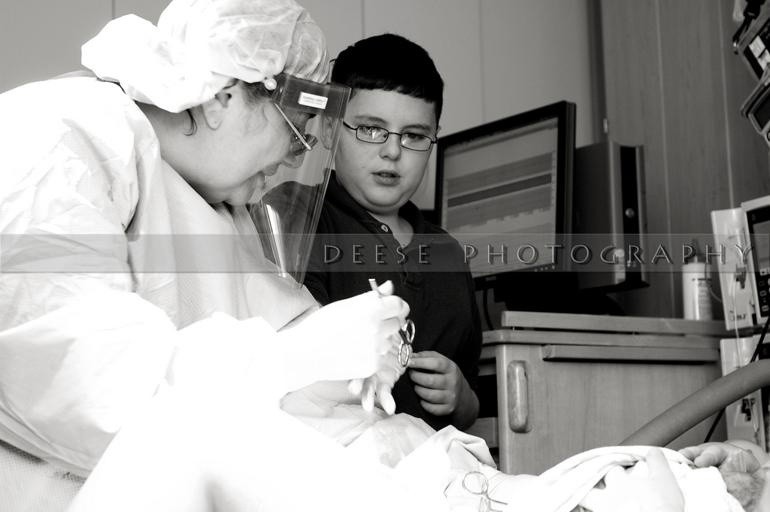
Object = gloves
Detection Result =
[346,315,416,416]
[275,276,407,399]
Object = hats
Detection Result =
[156,0,327,91]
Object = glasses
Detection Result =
[271,100,318,157]
[340,116,441,153]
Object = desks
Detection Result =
[462,310,727,476]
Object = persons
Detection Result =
[0,1,415,512]
[572,429,770,509]
[260,32,483,432]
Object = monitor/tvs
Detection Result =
[433,102,576,292]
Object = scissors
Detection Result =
[377,290,416,367]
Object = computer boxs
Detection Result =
[488,142,650,310]
